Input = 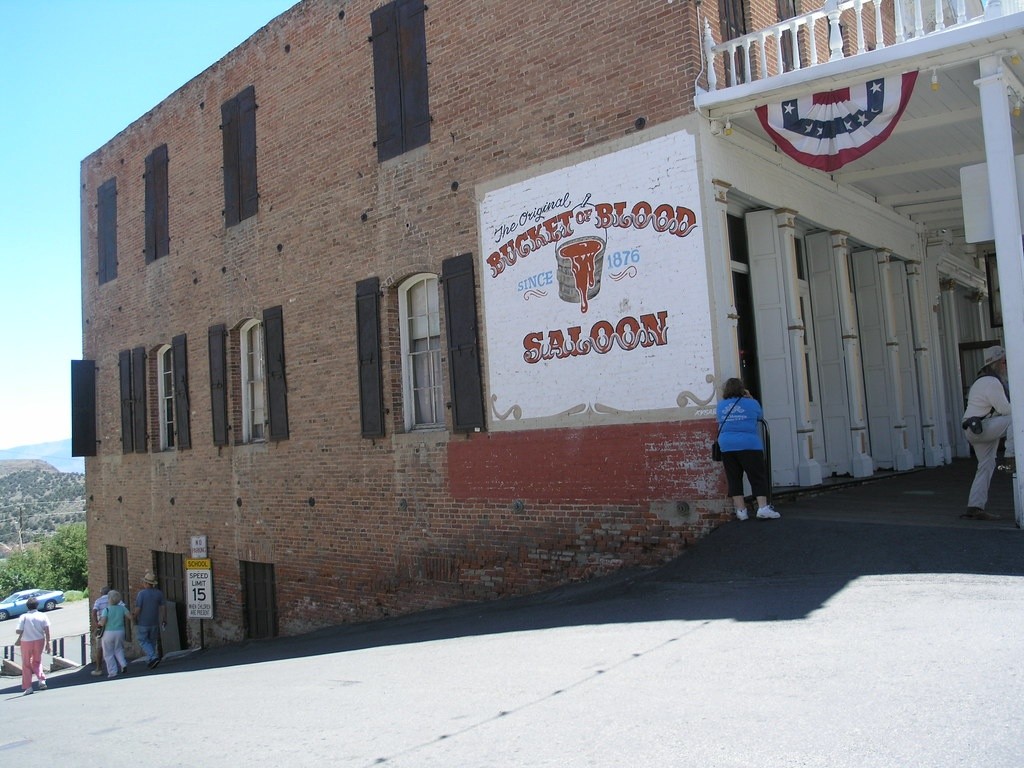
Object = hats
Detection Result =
[977,345,1005,375]
[140,573,158,585]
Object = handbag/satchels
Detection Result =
[711,442,722,461]
[95,607,108,638]
[15,615,26,646]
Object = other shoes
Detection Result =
[39,682,47,689]
[23,687,32,696]
[108,674,118,678]
[91,670,102,676]
[149,659,159,668]
[122,666,128,673]
[966,507,1000,520]
[1005,464,1016,473]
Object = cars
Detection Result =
[0,589,65,621]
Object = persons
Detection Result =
[91,587,127,675]
[98,590,135,677]
[134,573,168,669]
[717,378,780,521]
[16,598,51,696]
[962,346,1016,519]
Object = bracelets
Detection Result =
[47,641,50,643]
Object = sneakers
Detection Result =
[735,508,748,520]
[756,503,781,519]
